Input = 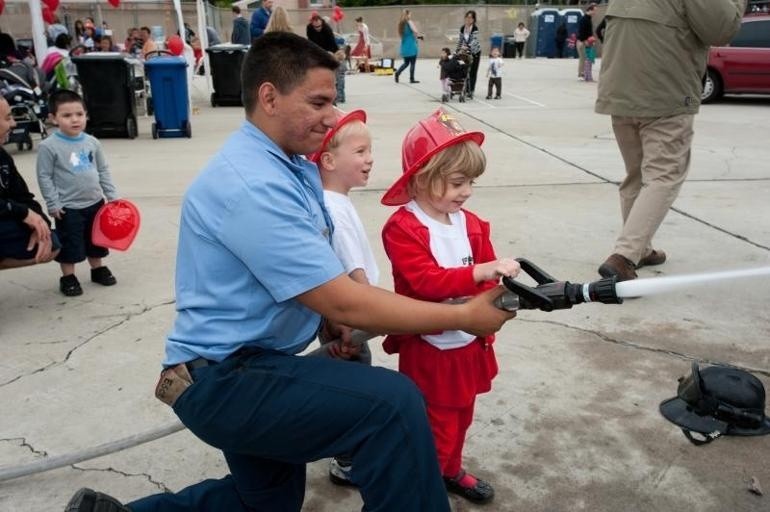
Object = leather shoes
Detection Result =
[441,466,496,506]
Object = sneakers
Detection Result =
[58,272,85,297]
[89,265,117,287]
[635,249,668,271]
[577,71,598,85]
[467,90,502,101]
[597,252,643,300]
[328,456,359,489]
[393,71,421,84]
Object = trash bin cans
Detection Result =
[489,34,516,58]
[71,52,191,140]
[205,43,245,108]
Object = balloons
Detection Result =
[168,35,184,55]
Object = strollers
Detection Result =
[0,74,46,154]
[437,46,476,104]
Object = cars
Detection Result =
[340,31,385,59]
[699,8,770,104]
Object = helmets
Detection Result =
[658,364,769,446]
[376,105,489,209]
[304,107,369,172]
[86,197,142,253]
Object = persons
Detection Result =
[231,0,289,45]
[0,93,61,270]
[304,108,377,486]
[438,11,482,101]
[64,29,518,512]
[36,89,117,297]
[206,25,222,47]
[394,8,421,83]
[565,3,607,84]
[177,22,195,44]
[593,1,746,280]
[307,16,340,54]
[335,49,347,103]
[380,106,522,506]
[41,13,157,92]
[356,17,370,46]
[485,46,505,99]
[514,22,531,59]
